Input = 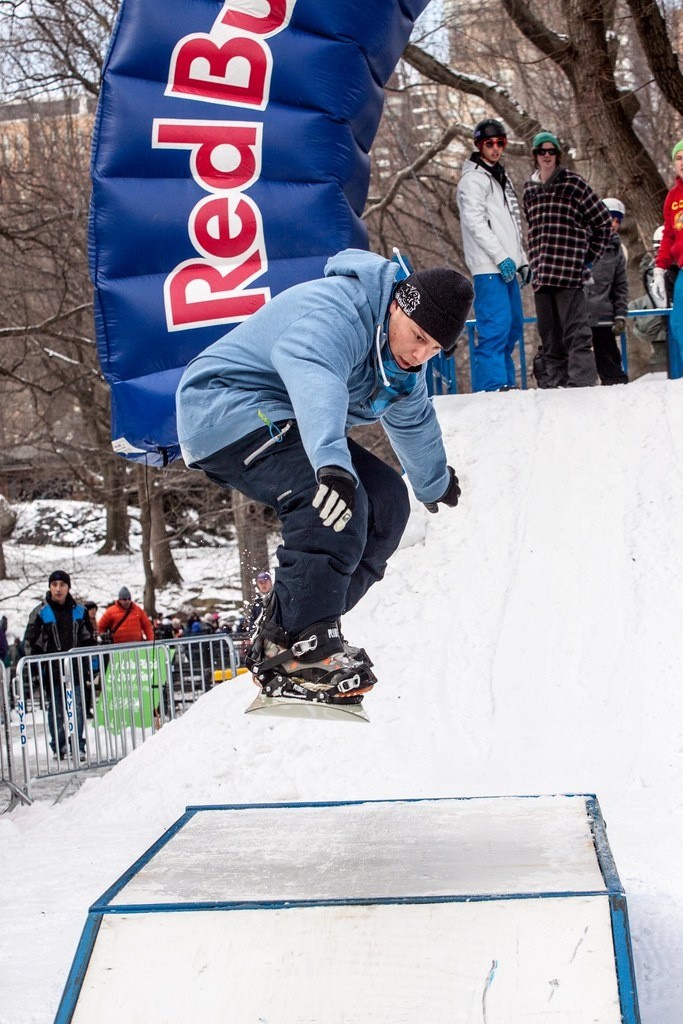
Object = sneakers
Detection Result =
[243,590,378,705]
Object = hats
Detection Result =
[162,619,170,625]
[394,269,474,351]
[85,601,97,610]
[119,587,131,599]
[213,614,220,620]
[671,140,683,160]
[172,618,181,628]
[532,132,559,149]
[219,621,227,628]
[258,572,271,581]
[49,570,70,588]
[204,613,214,620]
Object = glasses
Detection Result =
[481,139,504,148]
[120,598,130,601]
[537,148,557,155]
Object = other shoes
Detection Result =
[53,752,64,760]
[80,752,85,761]
[86,711,93,718]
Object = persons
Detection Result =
[651,141,683,379]
[98,587,154,643]
[522,132,612,389]
[23,570,102,760]
[175,248,476,723]
[249,572,273,630]
[84,600,109,718]
[455,119,534,394]
[585,198,629,386]
[150,612,240,717]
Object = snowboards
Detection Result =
[241,681,372,725]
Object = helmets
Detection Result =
[474,119,506,139]
[602,198,625,218]
[652,225,664,247]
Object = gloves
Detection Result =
[612,319,624,336]
[311,465,357,532]
[519,265,533,289]
[424,466,461,513]
[498,257,517,284]
[650,267,667,301]
